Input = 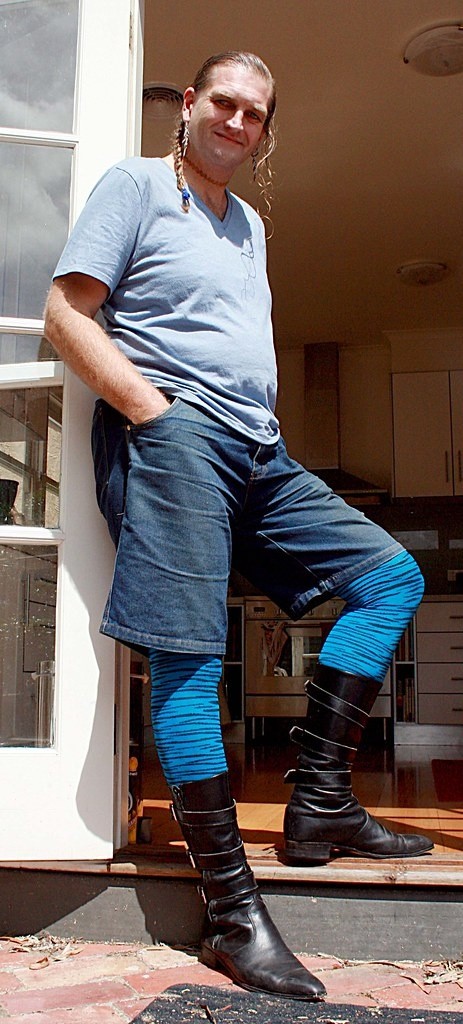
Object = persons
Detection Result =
[42,53,435,999]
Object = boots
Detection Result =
[282,662,434,860]
[169,770,327,1001]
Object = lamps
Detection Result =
[403,25,463,76]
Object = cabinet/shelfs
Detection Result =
[339,335,463,498]
[214,604,244,723]
[417,603,463,726]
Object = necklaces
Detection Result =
[184,156,229,187]
